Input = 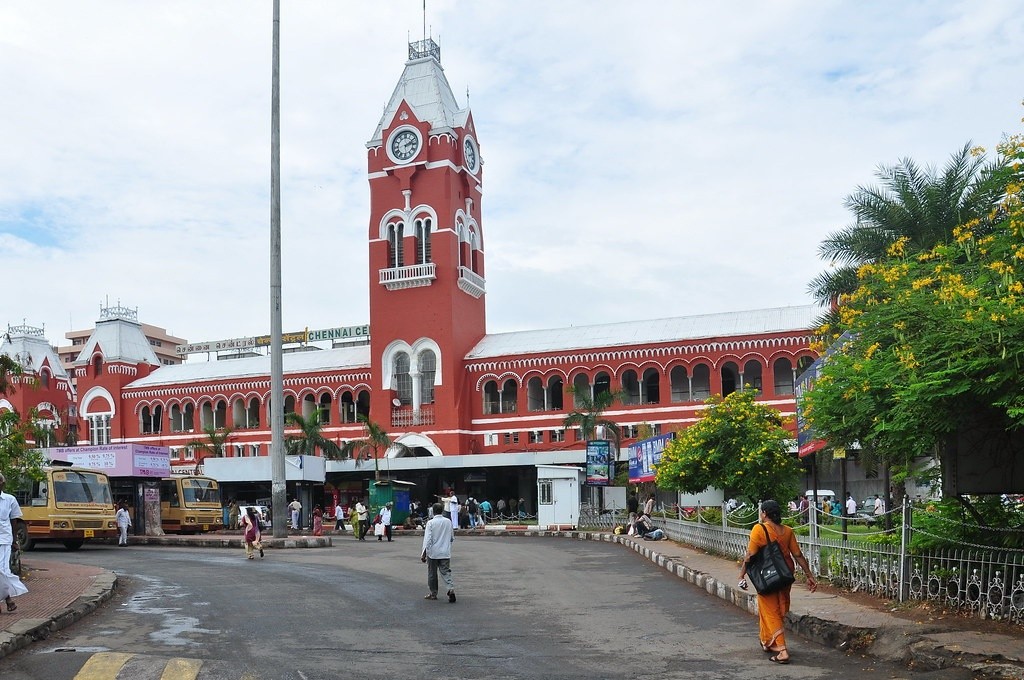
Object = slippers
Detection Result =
[760,640,771,651]
[770,656,790,664]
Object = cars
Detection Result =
[855,494,885,517]
[638,503,662,512]
[927,495,1024,504]
[673,502,703,516]
[726,498,754,513]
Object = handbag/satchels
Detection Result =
[745,523,795,595]
[375,523,384,535]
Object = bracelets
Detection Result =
[806,571,811,576]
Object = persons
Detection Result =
[739,500,817,664]
[348,499,370,541]
[0,473,28,614]
[378,503,395,543]
[497,497,506,516]
[227,499,241,530]
[313,504,322,536]
[627,491,655,538]
[335,502,346,530]
[788,495,809,525]
[479,499,492,521]
[873,494,882,515]
[437,491,478,530]
[421,503,456,603]
[823,496,842,517]
[846,492,857,518]
[509,497,518,515]
[240,507,264,560]
[288,498,302,529]
[724,495,737,512]
[403,499,434,530]
[116,503,132,547]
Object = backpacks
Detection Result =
[468,498,476,513]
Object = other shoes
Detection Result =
[633,534,642,538]
[260,548,264,557]
[388,539,394,542]
[5,598,16,611]
[447,590,456,603]
[249,556,254,559]
[119,543,128,547]
[425,594,437,600]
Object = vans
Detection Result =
[237,505,271,530]
[805,489,837,512]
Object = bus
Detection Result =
[1,458,118,551]
[107,474,223,535]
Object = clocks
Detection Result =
[385,124,423,165]
[463,134,480,175]
